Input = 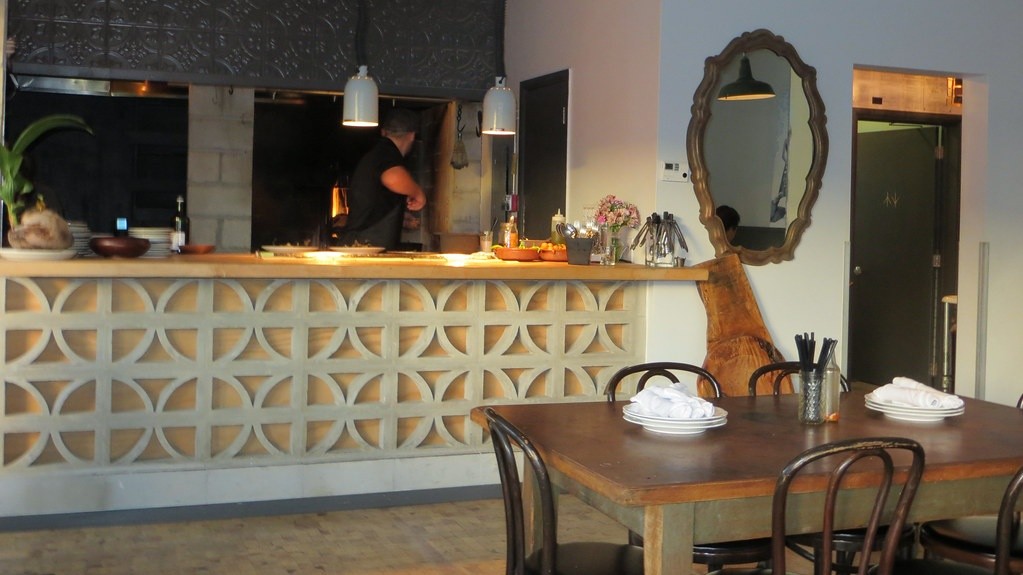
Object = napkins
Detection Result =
[630,383,715,418]
[872,376,960,408]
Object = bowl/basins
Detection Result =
[89,237,152,257]
[494,240,568,262]
[179,246,215,254]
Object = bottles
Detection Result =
[825,342,840,421]
[171,194,190,253]
[551,209,566,244]
[498,223,518,248]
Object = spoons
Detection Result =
[556,223,577,239]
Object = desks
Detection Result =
[470,391,1023,575]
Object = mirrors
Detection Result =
[689,33,828,266]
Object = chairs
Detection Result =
[484,407,644,575]
[705,438,925,574]
[603,362,773,573]
[918,468,1023,575]
[749,362,918,575]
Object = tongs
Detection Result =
[630,212,688,259]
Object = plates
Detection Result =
[126,229,173,257]
[864,394,965,422]
[67,223,92,257]
[623,404,728,435]
[0,248,77,260]
[262,246,319,256]
[329,247,385,258]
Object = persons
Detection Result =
[346,107,427,250]
[717,206,740,243]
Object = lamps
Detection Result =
[716,52,776,100]
[342,0,379,127]
[480,1,518,136]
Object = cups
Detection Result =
[646,224,674,268]
[480,231,493,253]
[566,238,593,265]
[799,372,825,425]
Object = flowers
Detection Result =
[592,193,640,229]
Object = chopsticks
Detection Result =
[795,332,838,372]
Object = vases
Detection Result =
[606,225,622,264]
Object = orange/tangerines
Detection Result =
[541,242,567,250]
